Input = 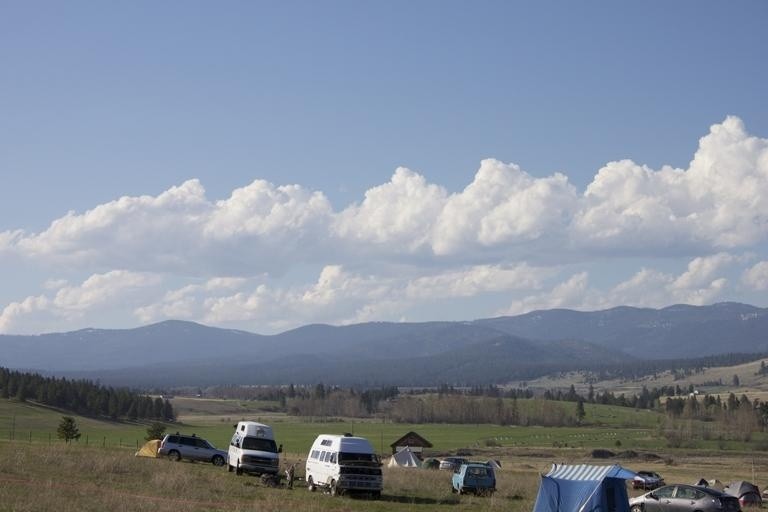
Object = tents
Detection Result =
[437,455,470,472]
[423,458,440,469]
[135,439,163,458]
[694,478,710,487]
[725,480,762,508]
[533,462,642,512]
[387,445,422,468]
[707,478,725,492]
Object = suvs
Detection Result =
[157,435,229,468]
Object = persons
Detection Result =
[258,464,296,489]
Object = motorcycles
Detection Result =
[285,458,301,489]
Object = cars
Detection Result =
[451,463,497,498]
[634,471,665,491]
[630,484,740,511]
[440,457,468,472]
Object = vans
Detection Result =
[228,422,283,478]
[305,434,383,500]
